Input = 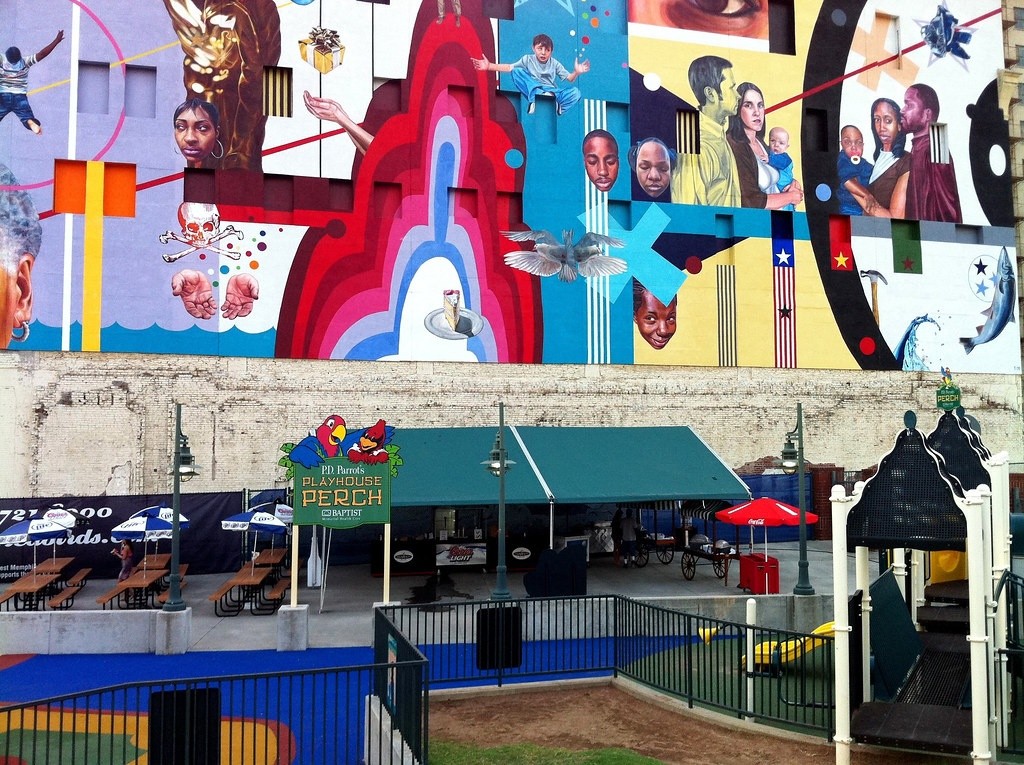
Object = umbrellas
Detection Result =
[111,506,190,578]
[716,497,818,593]
[6,506,92,584]
[222,497,293,576]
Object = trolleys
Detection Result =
[615,500,682,567]
[678,499,741,587]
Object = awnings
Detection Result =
[307,426,753,587]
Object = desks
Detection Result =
[230,548,289,615]
[6,558,75,611]
[119,552,171,610]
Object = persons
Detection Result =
[470,34,590,116]
[173,99,221,168]
[633,278,677,350]
[0,27,65,134]
[850,98,914,219]
[114,542,131,584]
[303,90,375,156]
[835,125,874,215]
[437,0,462,28]
[172,269,259,321]
[582,129,619,192]
[726,82,804,211]
[0,164,42,350]
[628,138,677,198]
[670,56,742,208]
[899,84,962,224]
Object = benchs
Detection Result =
[0,569,92,611]
[208,559,304,617]
[96,564,188,610]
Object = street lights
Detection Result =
[479,401,519,599]
[160,403,204,612]
[774,402,817,596]
